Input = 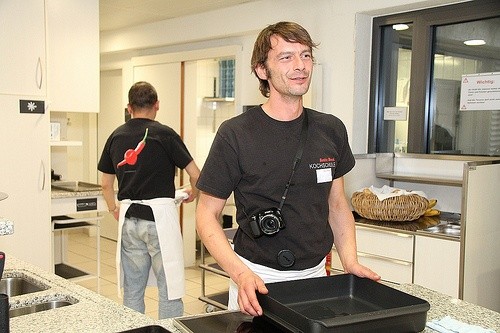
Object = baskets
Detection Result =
[351,188,429,222]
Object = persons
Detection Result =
[98,81,200,319]
[195,22,381,317]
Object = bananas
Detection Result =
[422,217,440,227]
[425,200,440,216]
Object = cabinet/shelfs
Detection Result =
[0,0,102,295]
[328,150,500,313]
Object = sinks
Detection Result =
[0,272,52,298]
[7,294,79,319]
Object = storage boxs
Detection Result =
[255,275,432,333]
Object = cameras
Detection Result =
[249,207,287,239]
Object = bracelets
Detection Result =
[110,206,116,211]
